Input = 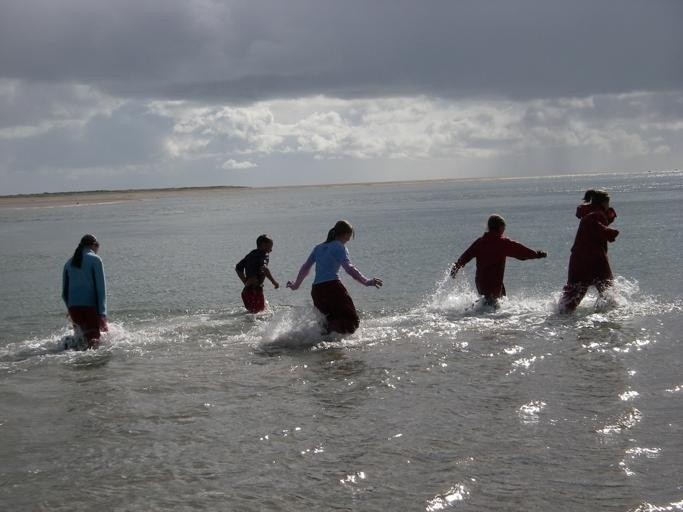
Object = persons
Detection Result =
[233,233,281,313]
[286,220,384,335]
[449,212,549,312]
[60,233,109,347]
[559,188,620,313]
